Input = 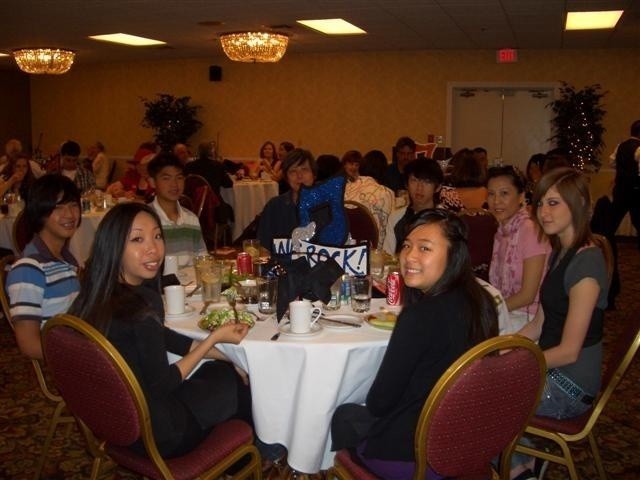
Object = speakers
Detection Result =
[208,65,222,82]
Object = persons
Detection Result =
[444,148,487,208]
[0,152,36,214]
[543,154,566,175]
[527,152,545,183]
[483,165,552,336]
[492,168,613,480]
[146,152,209,269]
[297,149,408,250]
[263,142,296,181]
[610,120,640,250]
[67,203,289,480]
[92,141,109,188]
[314,154,341,183]
[5,173,82,361]
[183,143,233,224]
[340,151,362,180]
[251,141,281,178]
[383,158,450,254]
[0,140,41,179]
[473,147,488,183]
[386,137,416,190]
[331,208,498,480]
[175,144,194,168]
[546,149,622,311]
[254,147,332,251]
[50,140,94,195]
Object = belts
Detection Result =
[546,367,598,405]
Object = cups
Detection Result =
[243,239,259,262]
[324,276,343,311]
[216,261,234,286]
[162,285,184,314]
[350,275,373,312]
[256,276,278,314]
[199,264,222,303]
[193,256,214,287]
[290,301,321,333]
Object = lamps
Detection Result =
[296,17,368,36]
[89,31,167,46]
[562,10,623,29]
[11,48,76,75]
[218,30,288,63]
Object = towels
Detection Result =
[305,258,345,305]
[272,253,292,266]
[271,273,290,341]
[287,257,310,300]
[300,261,323,302]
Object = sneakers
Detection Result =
[535,446,552,480]
[512,469,534,480]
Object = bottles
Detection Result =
[237,252,252,276]
[386,272,402,306]
[83,198,91,214]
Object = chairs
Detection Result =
[343,199,378,247]
[107,160,116,183]
[1,254,99,453]
[331,334,546,480]
[42,314,262,480]
[189,185,207,216]
[188,176,232,248]
[524,329,640,478]
[457,209,498,267]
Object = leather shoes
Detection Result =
[254,435,286,462]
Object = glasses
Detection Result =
[288,167,308,175]
[409,176,433,185]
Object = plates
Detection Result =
[363,310,401,330]
[320,314,364,329]
[163,304,197,318]
[184,286,201,294]
[278,321,324,336]
[197,311,257,334]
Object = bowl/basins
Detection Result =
[233,280,264,298]
